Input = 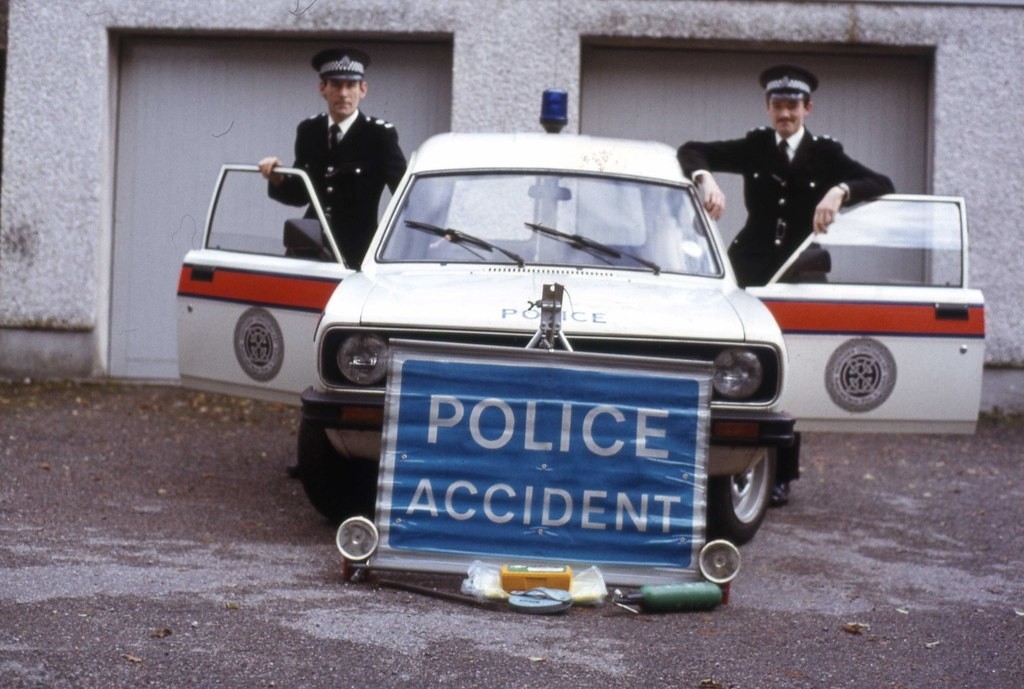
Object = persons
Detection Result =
[258,47,408,478]
[677,63,895,507]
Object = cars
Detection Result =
[177,91,987,545]
